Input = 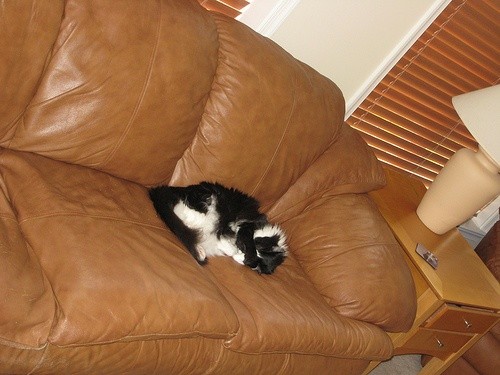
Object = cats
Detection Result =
[148,180,288,275]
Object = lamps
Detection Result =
[415,83,499,235]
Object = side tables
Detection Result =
[364,164,499,375]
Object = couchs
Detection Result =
[0,1,418,373]
